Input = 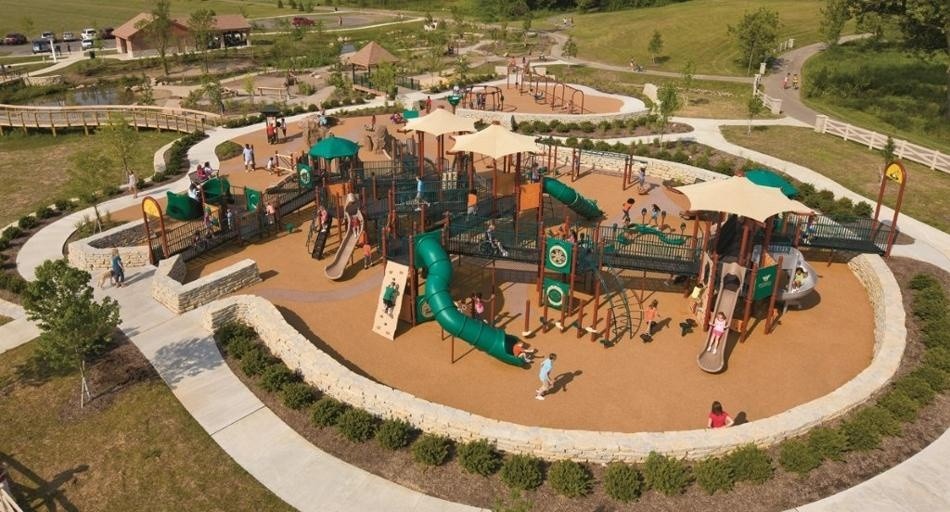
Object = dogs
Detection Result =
[97,268,125,289]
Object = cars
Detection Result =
[291,17,316,27]
[1,26,114,53]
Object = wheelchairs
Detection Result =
[479,231,504,258]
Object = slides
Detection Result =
[412,230,530,367]
[324,193,365,280]
[697,262,746,372]
[542,177,602,220]
[266,172,296,193]
[773,247,816,308]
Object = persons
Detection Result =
[535,352,557,401]
[109,247,128,288]
[473,291,496,314]
[4,65,11,78]
[689,283,705,310]
[67,44,72,54]
[338,16,344,26]
[783,71,799,89]
[313,175,427,318]
[318,57,533,131]
[627,58,642,71]
[469,163,659,259]
[191,119,286,252]
[126,170,139,198]
[792,268,806,292]
[646,298,661,341]
[707,312,729,354]
[708,400,734,428]
[513,341,534,364]
[562,15,575,27]
